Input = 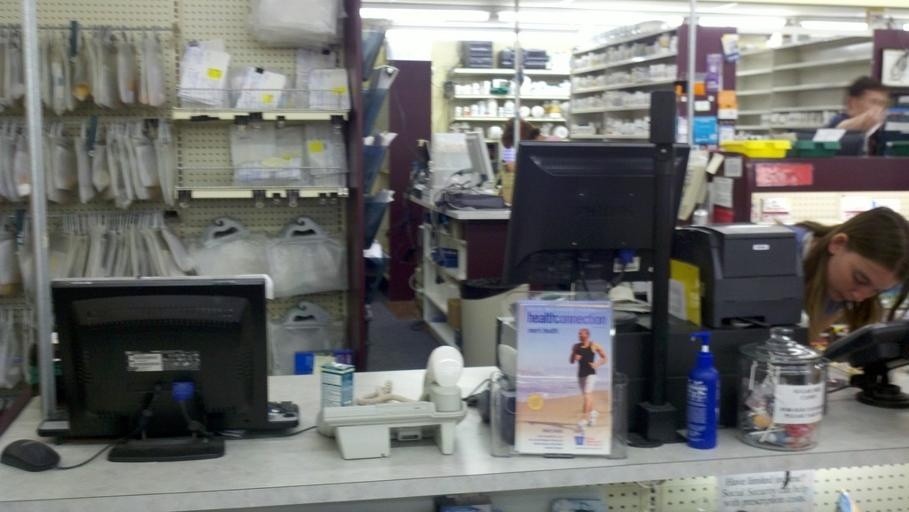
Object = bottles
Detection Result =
[736,327,826,452]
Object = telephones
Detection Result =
[317,346,466,459]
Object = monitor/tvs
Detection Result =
[466,132,495,192]
[502,139,689,305]
[50,276,268,462]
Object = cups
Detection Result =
[500,171,515,204]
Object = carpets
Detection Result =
[387,299,423,322]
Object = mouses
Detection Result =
[0,439,59,471]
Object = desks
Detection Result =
[1,358,909,512]
[414,187,513,353]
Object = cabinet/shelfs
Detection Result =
[172,108,351,205]
[363,17,401,304]
[449,22,909,154]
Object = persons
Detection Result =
[772,206,909,350]
[569,327,608,428]
[822,75,894,133]
[500,115,564,174]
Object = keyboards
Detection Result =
[39,401,299,436]
